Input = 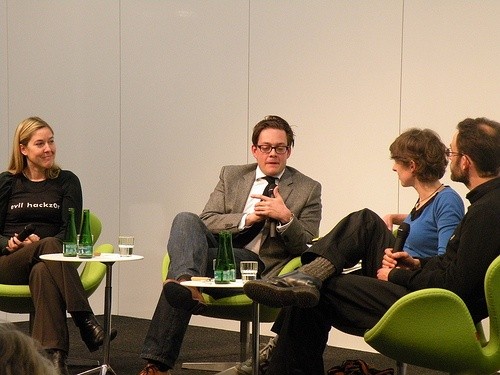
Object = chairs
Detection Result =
[162,252,302,375]
[366,230,500,375]
[0,214,114,337]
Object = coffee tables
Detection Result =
[39,253,144,375]
[180,279,259,375]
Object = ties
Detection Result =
[232,174,277,249]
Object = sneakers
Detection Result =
[236,337,277,375]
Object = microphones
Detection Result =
[392,222,411,254]
[2,223,37,254]
[268,183,278,237]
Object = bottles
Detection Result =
[78,209,94,259]
[214,230,236,284]
[62,208,78,257]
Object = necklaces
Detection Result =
[416,185,444,207]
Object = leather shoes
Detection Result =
[162,278,209,315]
[137,362,173,375]
[243,268,322,309]
[80,322,117,352]
[45,348,70,375]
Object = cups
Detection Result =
[118,236,134,257]
[76,234,93,246]
[240,261,258,284]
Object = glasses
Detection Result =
[254,144,289,155]
[445,148,472,165]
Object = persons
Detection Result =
[140,116,322,375]
[244,118,500,375]
[0,324,59,375]
[0,116,118,375]
[380,128,465,259]
